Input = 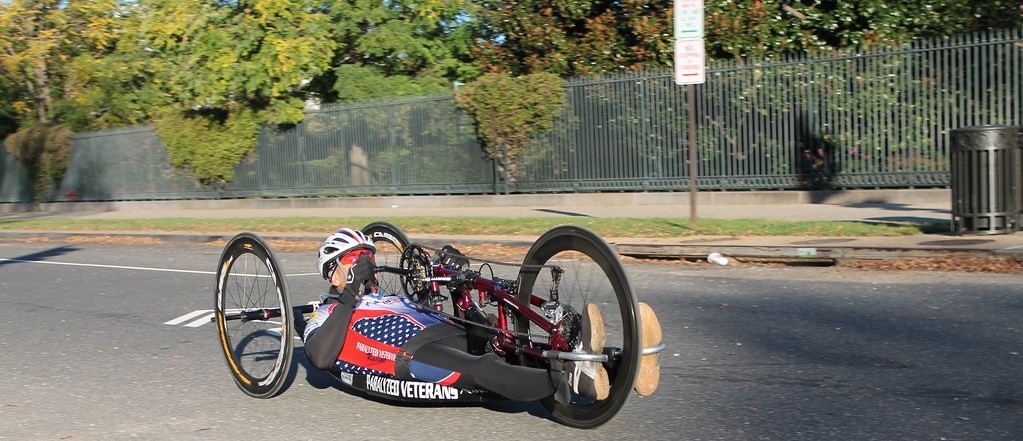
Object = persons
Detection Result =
[303,228,663,402]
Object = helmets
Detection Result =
[316,227,376,279]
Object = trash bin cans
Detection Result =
[947,125,1022,235]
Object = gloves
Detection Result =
[440,244,470,272]
[338,254,375,301]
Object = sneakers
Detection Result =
[634,302,662,396]
[570,302,609,399]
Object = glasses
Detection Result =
[340,249,375,264]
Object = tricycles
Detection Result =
[210,221,667,431]
